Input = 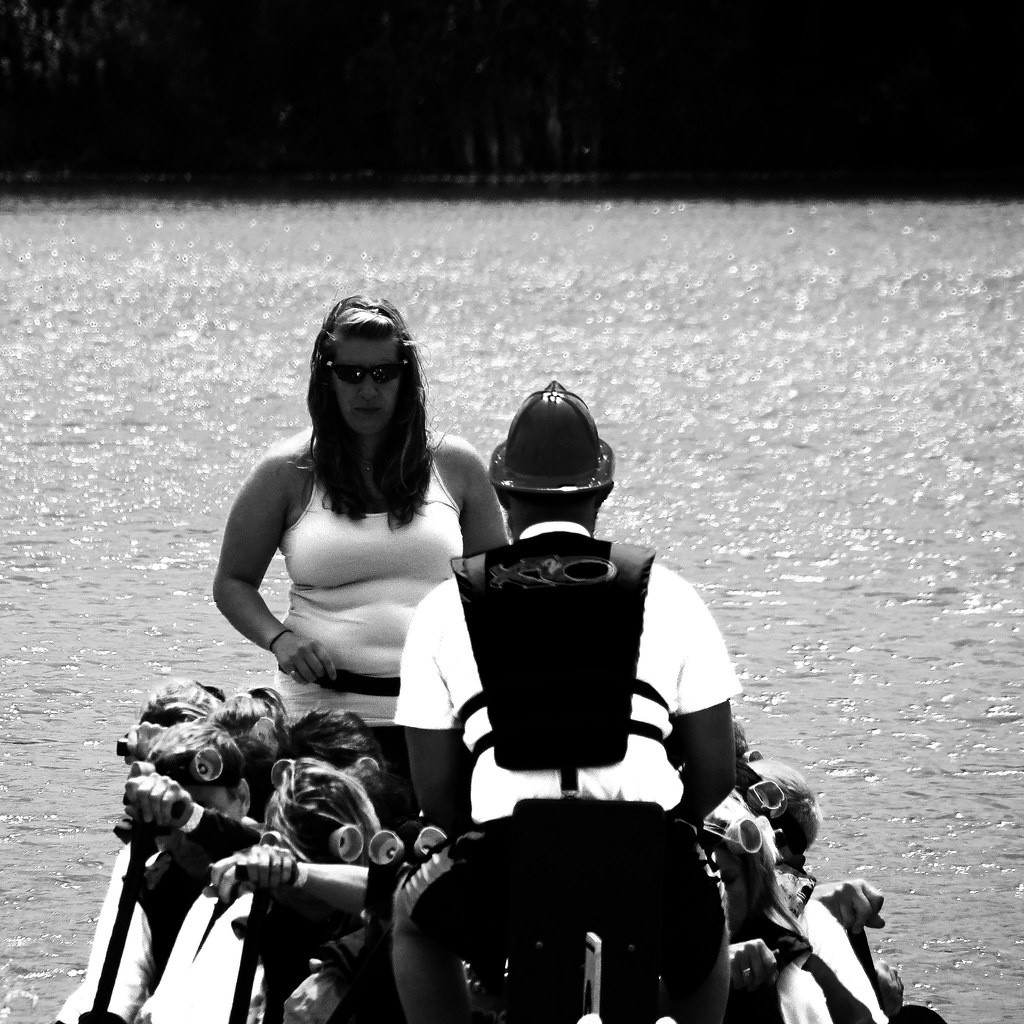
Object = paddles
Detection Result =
[227,860,298,1024]
[88,790,187,1024]
[846,926,947,1024]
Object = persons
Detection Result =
[55,679,424,1024]
[392,378,740,1023]
[693,719,905,1024]
[210,292,512,829]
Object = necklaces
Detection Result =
[362,458,373,470]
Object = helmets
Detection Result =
[487,381,614,493]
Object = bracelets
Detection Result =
[268,629,294,652]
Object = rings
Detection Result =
[289,669,296,676]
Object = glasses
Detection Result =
[328,363,404,384]
